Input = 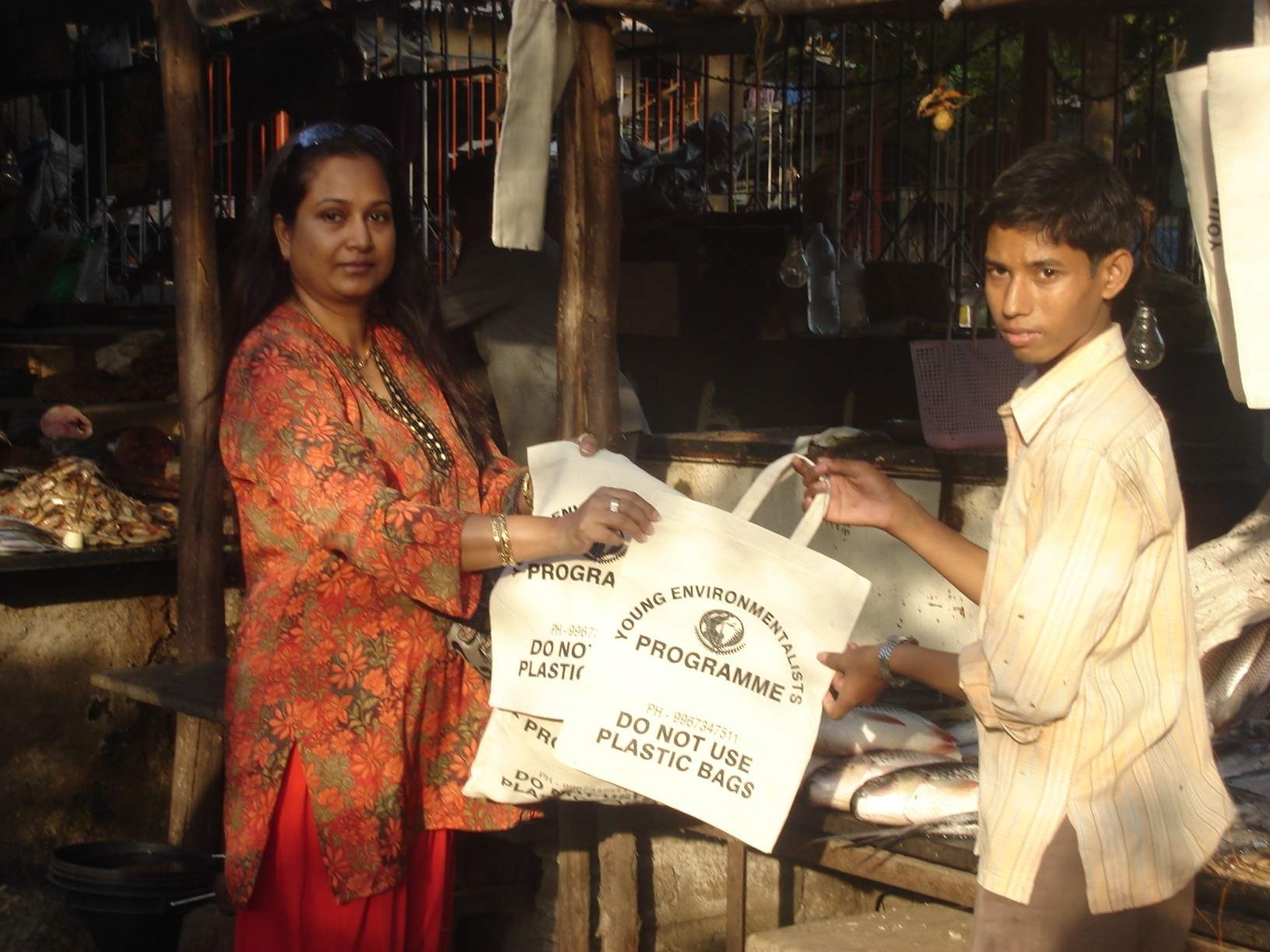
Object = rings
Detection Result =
[579,432,597,445]
[610,497,620,512]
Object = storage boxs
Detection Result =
[615,259,681,337]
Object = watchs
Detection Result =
[878,635,919,686]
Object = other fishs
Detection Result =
[1198,616,1269,740]
[801,703,981,868]
[1,516,72,557]
[3,465,38,474]
[0,473,20,482]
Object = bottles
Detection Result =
[959,256,988,338]
[805,223,840,334]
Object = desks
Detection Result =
[79,657,1270,952]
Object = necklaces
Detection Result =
[355,347,372,369]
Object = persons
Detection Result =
[792,146,1240,952]
[221,119,663,951]
[432,154,653,470]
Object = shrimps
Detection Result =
[0,455,181,546]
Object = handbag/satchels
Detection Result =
[490,442,687,721]
[461,708,669,805]
[551,451,874,856]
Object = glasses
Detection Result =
[288,125,396,170]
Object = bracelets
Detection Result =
[491,512,517,568]
[523,472,534,511]
